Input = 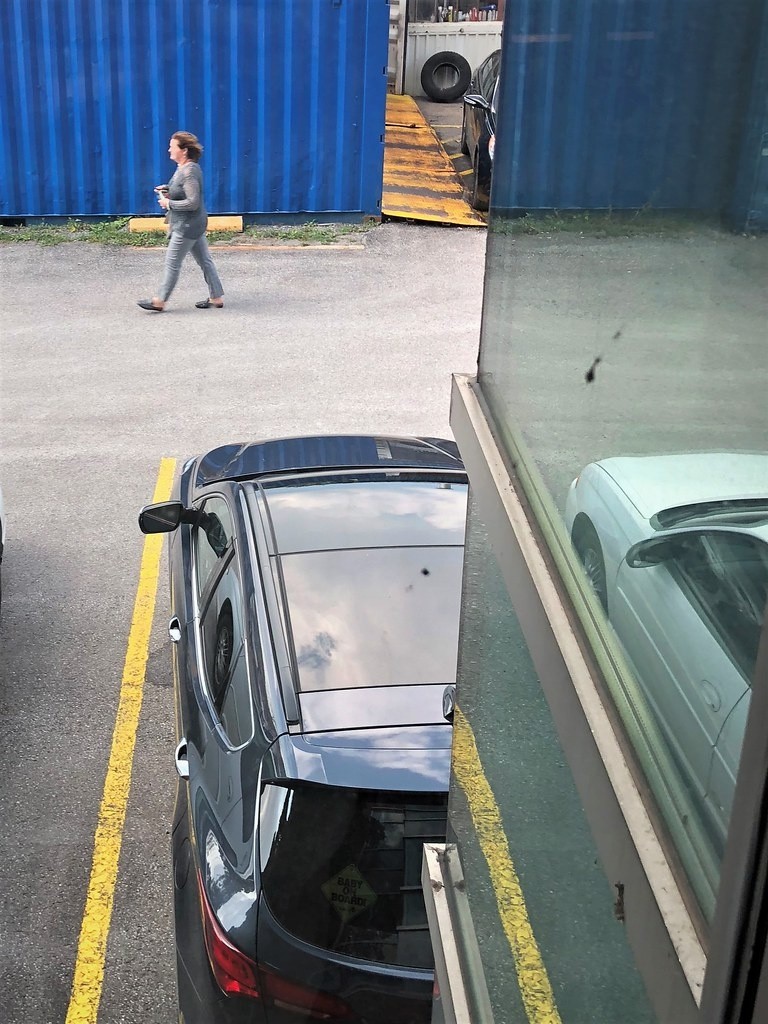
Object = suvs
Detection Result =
[140,433,483,1024]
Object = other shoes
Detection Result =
[195,298,223,308]
[137,299,163,312]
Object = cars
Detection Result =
[458,46,504,210]
[567,445,767,863]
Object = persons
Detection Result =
[136,132,224,310]
